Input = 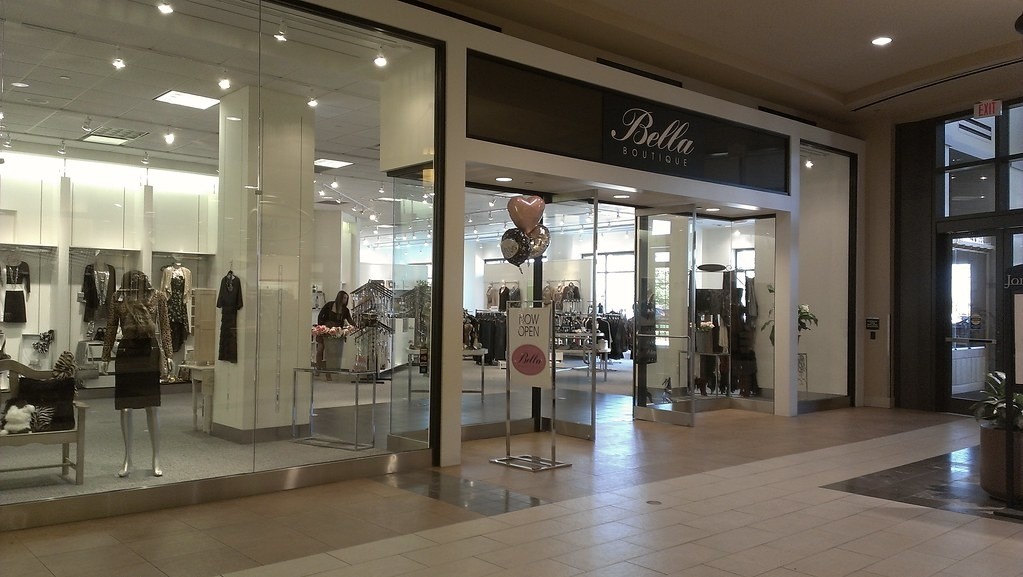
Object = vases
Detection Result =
[323,337,345,371]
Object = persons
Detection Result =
[543,283,580,307]
[315,290,355,381]
[160,263,192,352]
[83,261,116,322]
[486,284,520,310]
[100,271,175,477]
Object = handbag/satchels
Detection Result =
[93,327,107,341]
[157,345,168,375]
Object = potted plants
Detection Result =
[969,370,1023,506]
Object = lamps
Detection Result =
[4,134,12,148]
[308,89,317,106]
[317,178,630,250]
[274,18,288,42]
[57,140,66,155]
[141,152,149,163]
[82,117,92,131]
[374,43,384,63]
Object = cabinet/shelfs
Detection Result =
[553,330,612,383]
[78,340,119,375]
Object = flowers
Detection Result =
[312,325,354,344]
[759,284,818,345]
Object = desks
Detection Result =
[177,364,214,435]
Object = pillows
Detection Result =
[18,377,75,433]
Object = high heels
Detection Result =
[661,391,673,404]
[662,377,671,390]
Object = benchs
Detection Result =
[0,359,91,485]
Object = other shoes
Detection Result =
[326,372,332,381]
[315,371,320,376]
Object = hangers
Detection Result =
[226,261,236,278]
[477,311,507,318]
[556,312,623,320]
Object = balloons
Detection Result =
[501,196,550,274]
